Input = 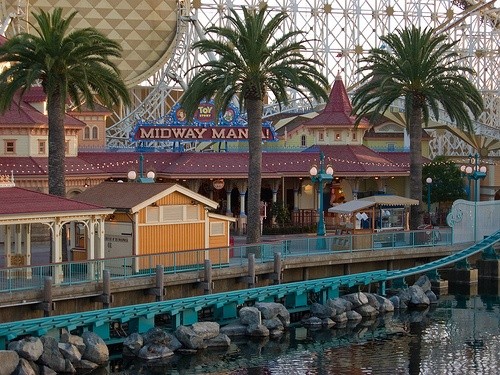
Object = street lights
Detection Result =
[467,153,487,244]
[426,177,433,213]
[309,149,333,249]
[462,155,480,203]
[130,155,153,184]
